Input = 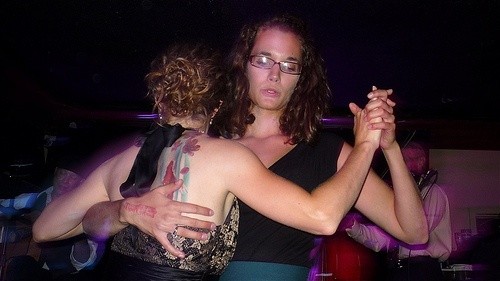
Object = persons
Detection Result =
[0,157,106,281]
[345,141,451,281]
[32,41,396,281]
[83,12,429,281]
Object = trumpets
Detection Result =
[411,167,439,200]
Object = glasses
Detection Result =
[247,55,304,75]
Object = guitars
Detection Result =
[0,215,43,263]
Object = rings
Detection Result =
[172,227,179,235]
